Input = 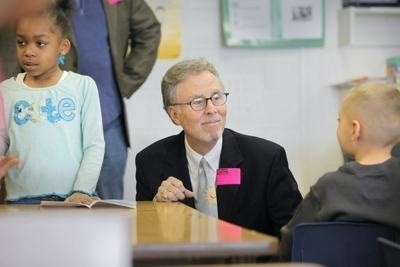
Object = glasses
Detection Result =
[168,93,229,111]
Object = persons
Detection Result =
[136,58,304,240]
[0,0,160,205]
[275,82,400,266]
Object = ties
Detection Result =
[198,158,216,216]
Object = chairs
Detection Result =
[291,221,393,267]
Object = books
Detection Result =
[40,199,135,210]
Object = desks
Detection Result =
[1,199,279,266]
